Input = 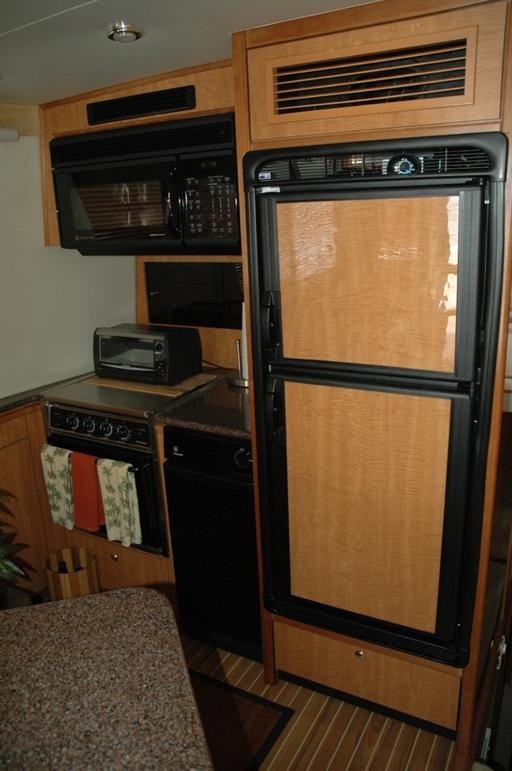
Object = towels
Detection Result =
[39,444,143,548]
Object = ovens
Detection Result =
[48,111,242,258]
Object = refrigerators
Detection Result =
[241,135,512,670]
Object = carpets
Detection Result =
[188,671,295,771]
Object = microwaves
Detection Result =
[92,320,203,387]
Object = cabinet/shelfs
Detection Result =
[65,529,169,593]
[271,622,460,742]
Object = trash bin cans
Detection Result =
[43,546,99,600]
